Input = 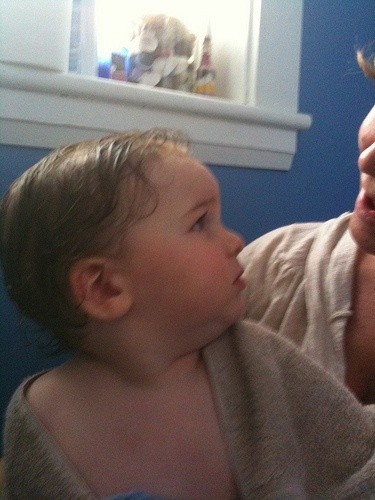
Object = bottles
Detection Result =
[194,32,219,94]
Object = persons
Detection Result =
[0,128,375,500]
[237,50,374,407]
